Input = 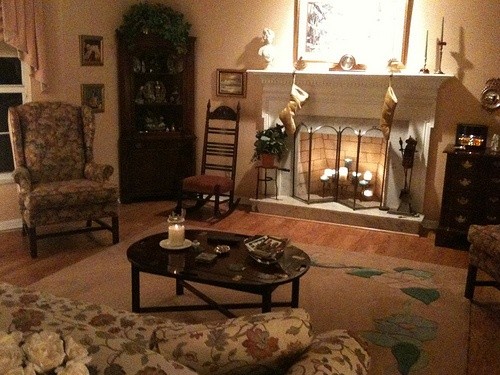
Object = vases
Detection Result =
[250,123,287,165]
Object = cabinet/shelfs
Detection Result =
[434,142,500,252]
[113,29,198,204]
[255,167,278,201]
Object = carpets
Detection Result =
[27,215,500,374]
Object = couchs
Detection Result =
[0,283,371,375]
[7,100,121,259]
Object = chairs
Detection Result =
[176,98,241,215]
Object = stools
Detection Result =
[464,224,500,300]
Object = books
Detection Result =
[246,235,268,252]
[253,236,286,259]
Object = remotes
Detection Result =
[207,234,242,246]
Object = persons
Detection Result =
[258,27,278,71]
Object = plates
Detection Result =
[160,239,193,250]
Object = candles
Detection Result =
[168,223,185,246]
[319,168,335,182]
[353,171,361,176]
[364,171,372,180]
[344,157,353,162]
[360,181,368,184]
[339,166,348,181]
[363,190,373,197]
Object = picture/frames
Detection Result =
[79,34,103,67]
[80,83,106,113]
[293,0,412,69]
[215,68,247,99]
